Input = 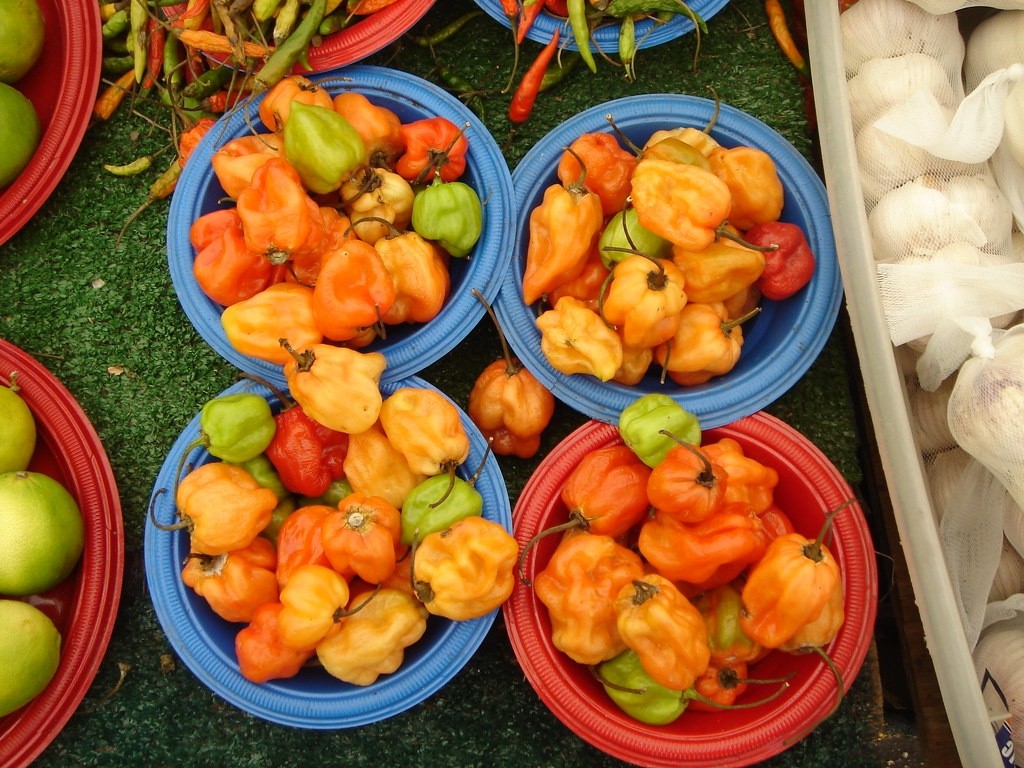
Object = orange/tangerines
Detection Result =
[0,386,85,719]
[0,0,44,188]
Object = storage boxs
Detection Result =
[805,0,1024,767]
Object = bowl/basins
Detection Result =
[140,0,441,78]
[489,91,841,431]
[1,0,103,251]
[496,401,876,768]
[142,365,514,730]
[0,336,125,768]
[165,66,515,389]
[468,0,732,55]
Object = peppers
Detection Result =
[92,0,845,719]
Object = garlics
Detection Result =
[833,0,1024,768]
